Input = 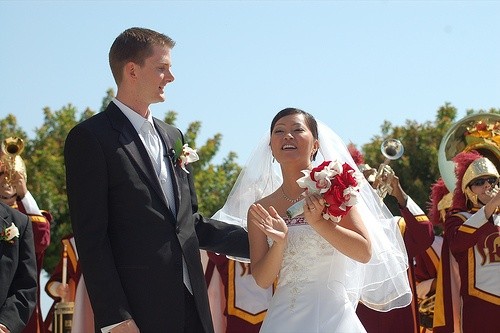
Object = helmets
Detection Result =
[454,151,500,204]
[429,178,454,226]
[0,155,27,183]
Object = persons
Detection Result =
[210,107,413,333]
[0,154,53,333]
[44,232,82,333]
[63,27,250,333]
[348,142,435,333]
[414,150,500,333]
[0,201,39,333]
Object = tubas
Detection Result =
[437,111,500,198]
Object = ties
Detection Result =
[141,120,161,165]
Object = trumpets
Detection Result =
[370,138,405,197]
[1,136,25,185]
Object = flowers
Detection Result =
[283,158,364,223]
[163,135,200,175]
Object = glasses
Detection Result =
[469,176,497,188]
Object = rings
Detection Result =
[311,207,315,211]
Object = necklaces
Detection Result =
[281,185,303,203]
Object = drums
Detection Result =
[51,301,75,333]
